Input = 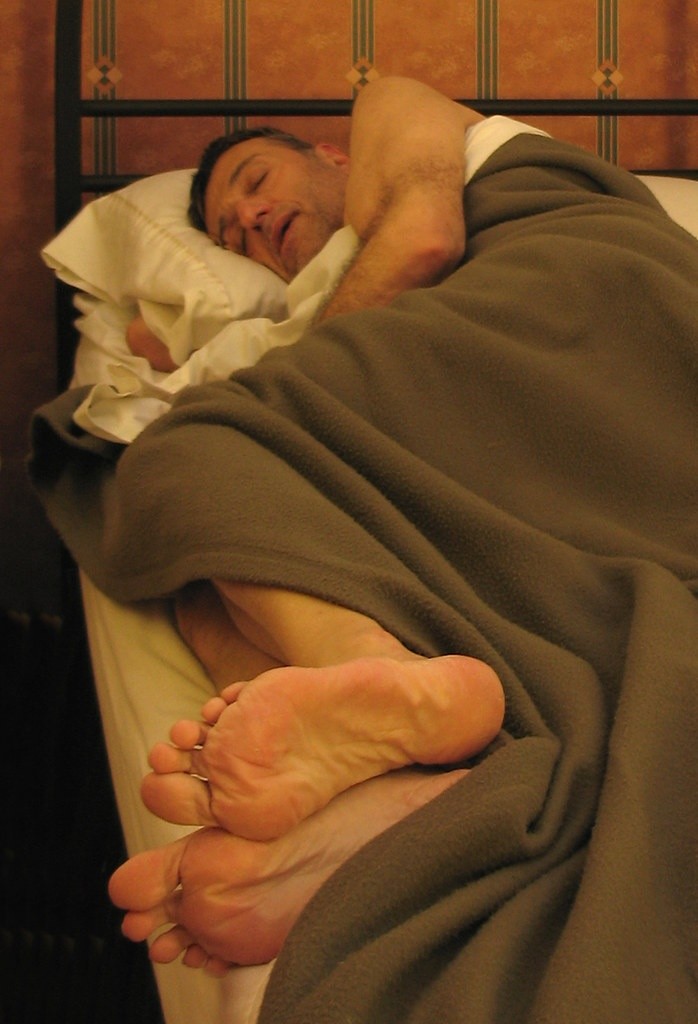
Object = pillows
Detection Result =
[40,166,698,366]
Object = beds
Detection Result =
[25,0,698,1024]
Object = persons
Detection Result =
[108,75,698,979]
